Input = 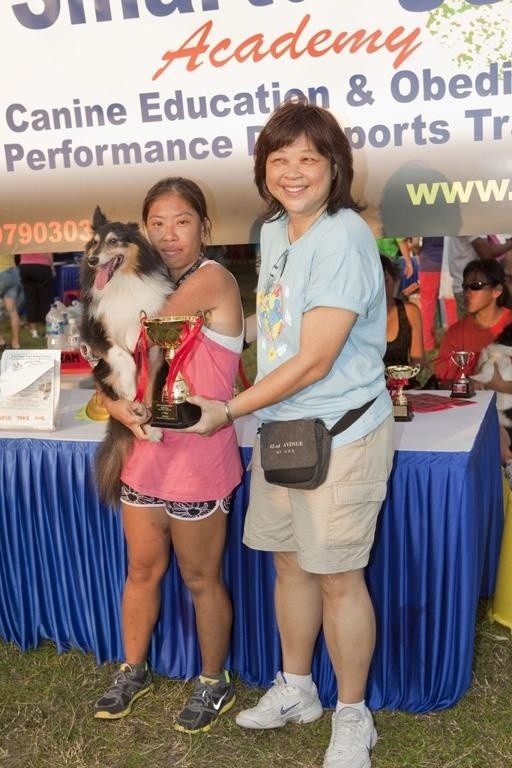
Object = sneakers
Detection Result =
[94,661,155,719]
[235,671,323,730]
[174,669,236,734]
[323,706,377,768]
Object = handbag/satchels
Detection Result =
[257,419,331,490]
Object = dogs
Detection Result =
[77,204,176,512]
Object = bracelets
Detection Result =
[224,400,236,424]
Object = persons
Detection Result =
[19,253,56,338]
[166,105,395,768]
[94,177,246,734]
[0,254,21,349]
[377,233,512,492]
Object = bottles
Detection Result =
[43,296,79,352]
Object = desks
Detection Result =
[1,387,502,713]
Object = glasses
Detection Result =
[266,250,287,298]
[462,281,486,291]
